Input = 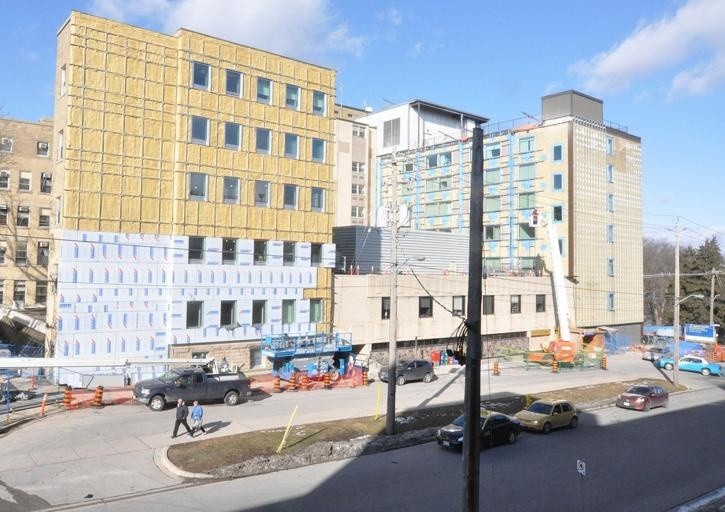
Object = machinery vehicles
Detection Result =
[527,218,585,367]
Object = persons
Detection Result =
[170,398,207,439]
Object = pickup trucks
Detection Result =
[131,367,252,411]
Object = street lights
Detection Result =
[673,294,705,386]
[386,255,426,435]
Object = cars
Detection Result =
[513,398,579,434]
[435,408,522,453]
[655,354,722,376]
[616,384,669,412]
[378,358,435,386]
[643,345,670,361]
